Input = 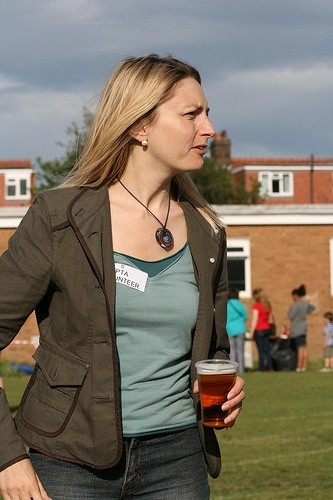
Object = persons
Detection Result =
[319,312,333,372]
[250,287,272,371]
[0,53,245,500]
[288,284,315,373]
[226,288,248,374]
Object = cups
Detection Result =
[195,359,240,427]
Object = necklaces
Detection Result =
[115,175,174,250]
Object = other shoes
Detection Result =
[319,368,333,372]
